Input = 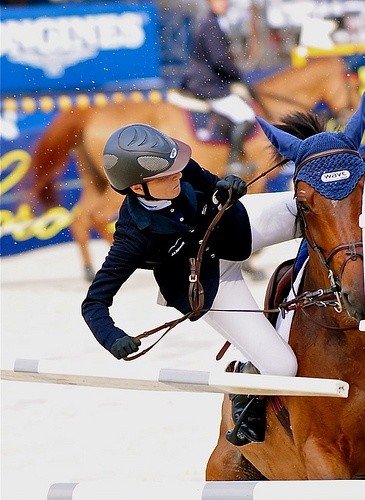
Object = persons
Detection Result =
[80,123,304,445]
[183,0,259,176]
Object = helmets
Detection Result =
[102,123,192,196]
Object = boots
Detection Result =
[226,361,266,442]
[229,120,257,175]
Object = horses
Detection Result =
[204,112,364,480]
[31,54,363,285]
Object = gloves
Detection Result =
[110,336,141,359]
[214,174,247,210]
